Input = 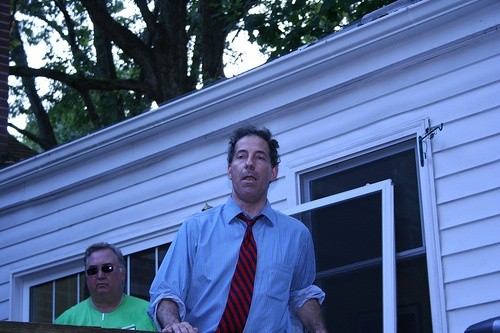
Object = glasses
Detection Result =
[85,265,121,275]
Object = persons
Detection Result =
[52,241,157,332]
[147,124,327,333]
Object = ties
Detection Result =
[212,213,263,333]
